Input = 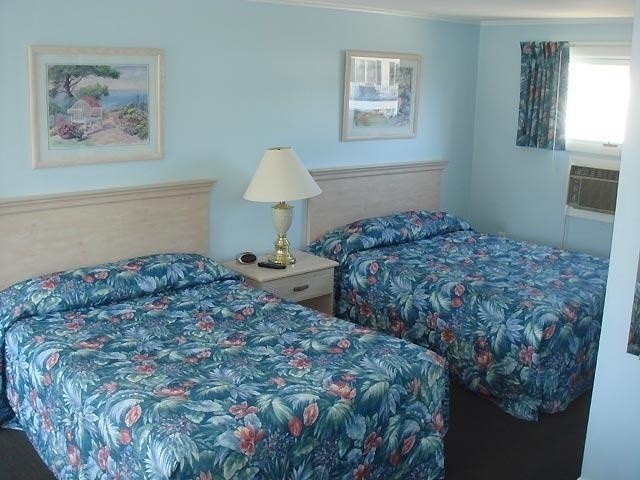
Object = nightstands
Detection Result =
[220,247,340,317]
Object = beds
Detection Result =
[306,160,639,423]
[0,178,450,480]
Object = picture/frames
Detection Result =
[340,49,423,142]
[27,44,167,170]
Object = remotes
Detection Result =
[257,261,287,269]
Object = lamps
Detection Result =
[241,146,323,266]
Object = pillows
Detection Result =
[0,253,244,321]
[303,209,475,262]
